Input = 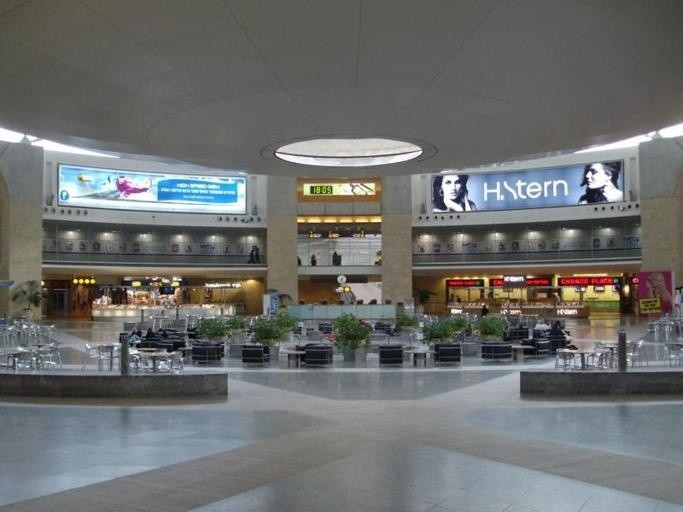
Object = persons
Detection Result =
[645,274,672,318]
[577,161,623,204]
[128,328,167,346]
[431,174,477,212]
[548,320,570,344]
[455,295,460,302]
[487,288,492,297]
[249,245,254,264]
[532,314,551,338]
[673,289,681,318]
[254,245,261,263]
[480,305,488,317]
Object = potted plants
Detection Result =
[249,311,296,362]
[330,313,373,362]
[448,312,508,356]
[9,277,44,323]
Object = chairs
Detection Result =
[555,311,682,368]
[0,314,121,371]
